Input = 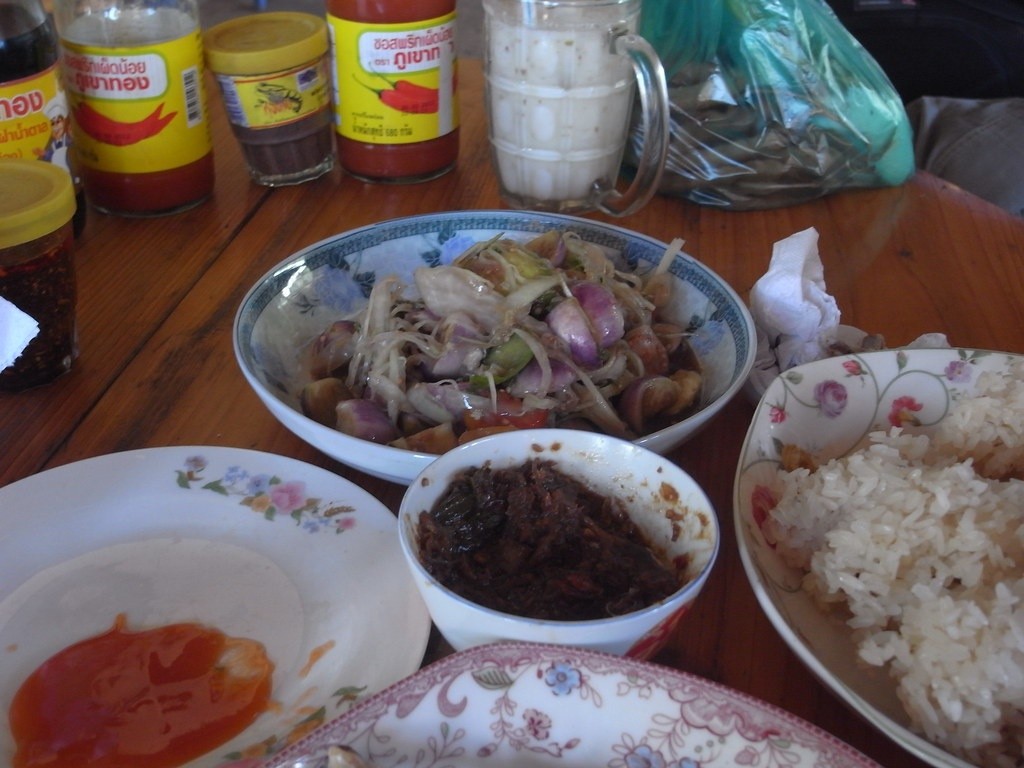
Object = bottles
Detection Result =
[46,0,215,218]
[0,151,77,382]
[200,11,335,187]
[1,0,86,239]
[320,0,459,186]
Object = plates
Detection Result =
[0,445,435,768]
[232,205,759,491]
[217,637,886,768]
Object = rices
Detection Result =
[757,379,1024,768]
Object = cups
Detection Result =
[478,1,669,218]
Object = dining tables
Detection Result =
[0,58,1024,768]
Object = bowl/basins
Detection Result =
[396,430,722,660]
[733,344,1024,768]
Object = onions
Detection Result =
[296,228,624,445]
